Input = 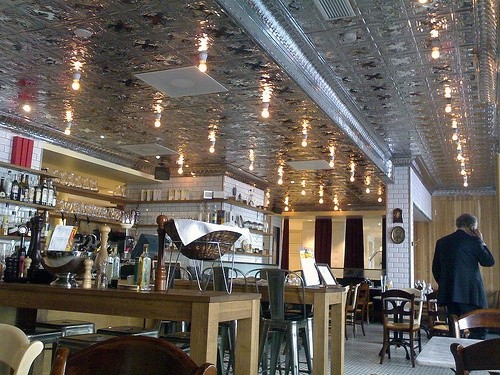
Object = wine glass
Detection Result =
[55,199,121,220]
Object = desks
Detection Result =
[0,282,262,375]
[151,281,350,375]
[374,296,439,360]
[415,336,500,373]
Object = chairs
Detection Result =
[346,275,500,375]
[51,335,217,375]
[0,323,44,375]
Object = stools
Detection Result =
[27,266,315,374]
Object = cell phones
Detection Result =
[471,226,475,232]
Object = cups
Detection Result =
[51,169,99,192]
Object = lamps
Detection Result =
[153,154,173,181]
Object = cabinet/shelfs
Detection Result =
[0,161,59,240]
[123,197,226,228]
[228,200,275,257]
[45,181,127,224]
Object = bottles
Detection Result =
[47,181,54,206]
[40,179,49,205]
[107,245,120,288]
[18,175,25,201]
[0,202,38,236]
[137,244,151,291]
[0,177,5,199]
[35,182,42,204]
[24,175,29,202]
[5,171,12,199]
[29,182,35,202]
[11,174,19,200]
[52,186,56,207]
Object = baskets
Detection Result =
[164,220,242,260]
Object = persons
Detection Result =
[432,213,495,340]
